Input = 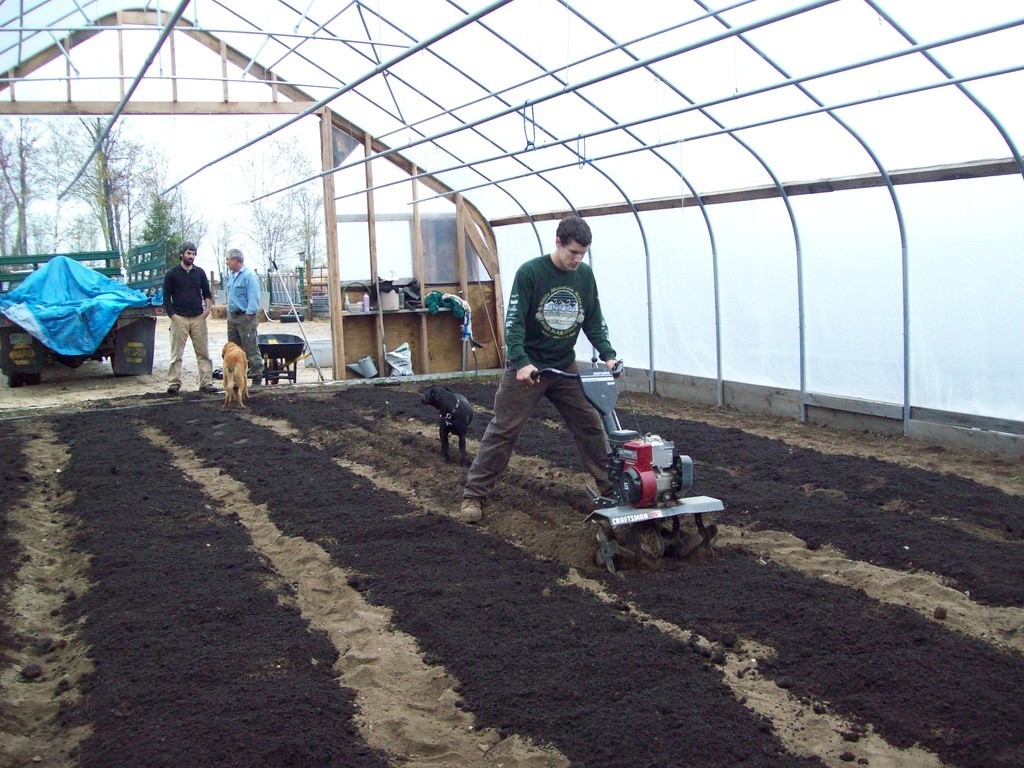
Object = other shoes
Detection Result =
[168,385,179,393]
[212,368,223,378]
[249,384,261,394]
[460,499,482,523]
[234,382,238,389]
[200,384,218,393]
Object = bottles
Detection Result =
[345,294,349,310]
[363,293,369,311]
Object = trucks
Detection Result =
[1,249,163,389]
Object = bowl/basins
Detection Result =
[348,304,362,312]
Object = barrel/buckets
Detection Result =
[303,338,332,367]
[381,289,399,311]
[312,295,329,320]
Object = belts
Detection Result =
[231,311,245,315]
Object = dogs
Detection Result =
[421,385,473,468]
[222,341,250,409]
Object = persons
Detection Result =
[163,243,218,391]
[461,214,625,523]
[220,249,263,393]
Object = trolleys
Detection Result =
[256,332,312,385]
